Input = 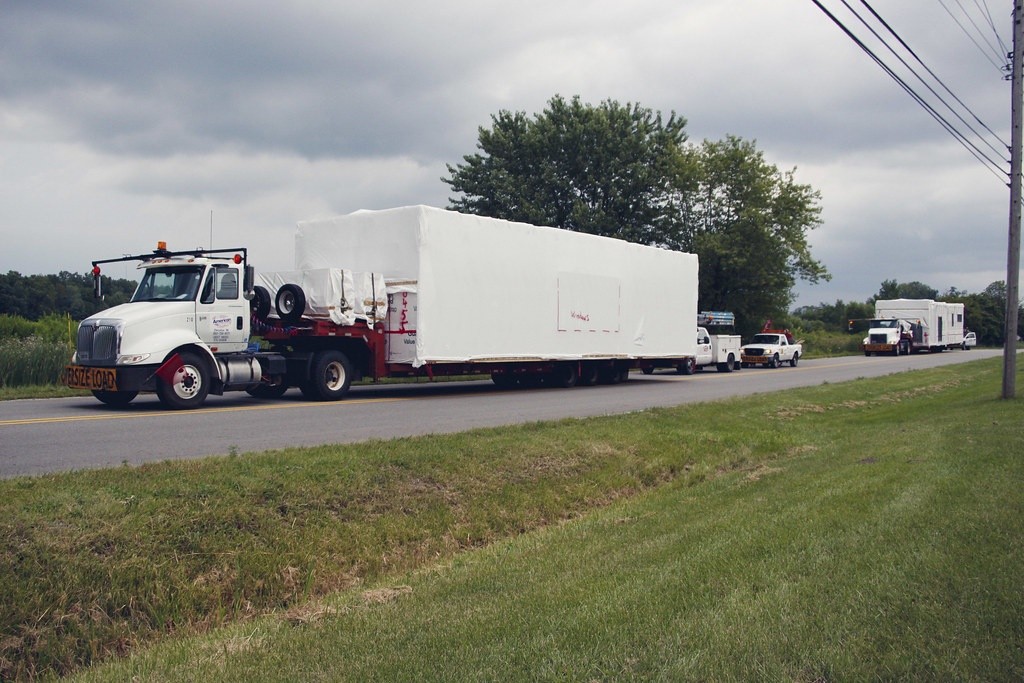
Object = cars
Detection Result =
[950,330,976,350]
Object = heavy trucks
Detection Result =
[61,204,698,410]
[863,299,964,357]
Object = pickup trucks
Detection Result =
[740,334,802,369]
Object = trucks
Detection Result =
[639,327,741,375]
